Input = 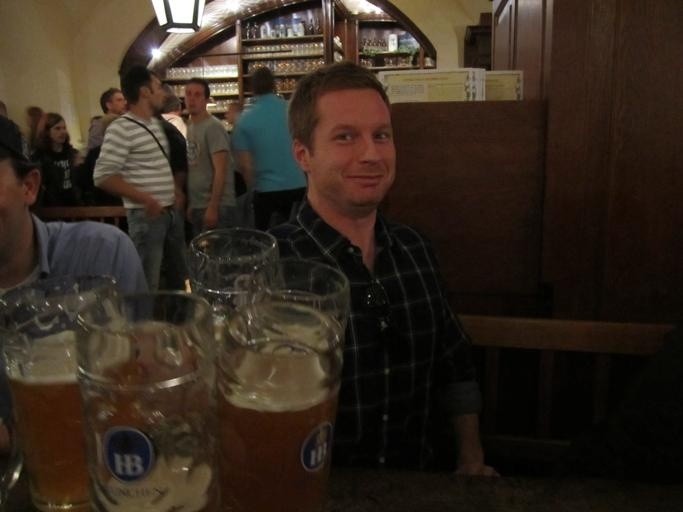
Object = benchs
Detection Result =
[35,204,129,233]
[455,316,683,479]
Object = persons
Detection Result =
[94,63,187,319]
[232,65,307,256]
[224,103,246,199]
[1,81,128,233]
[0,114,152,457]
[155,82,191,206]
[185,77,237,263]
[263,62,501,492]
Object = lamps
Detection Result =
[152,0,205,34]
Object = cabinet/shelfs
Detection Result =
[489,0,683,327]
[117,0,437,129]
[462,22,490,69]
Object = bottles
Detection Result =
[165,12,407,132]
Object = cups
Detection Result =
[214,303,353,511]
[1,274,115,510]
[71,290,220,510]
[187,228,351,374]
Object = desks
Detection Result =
[325,464,683,512]
